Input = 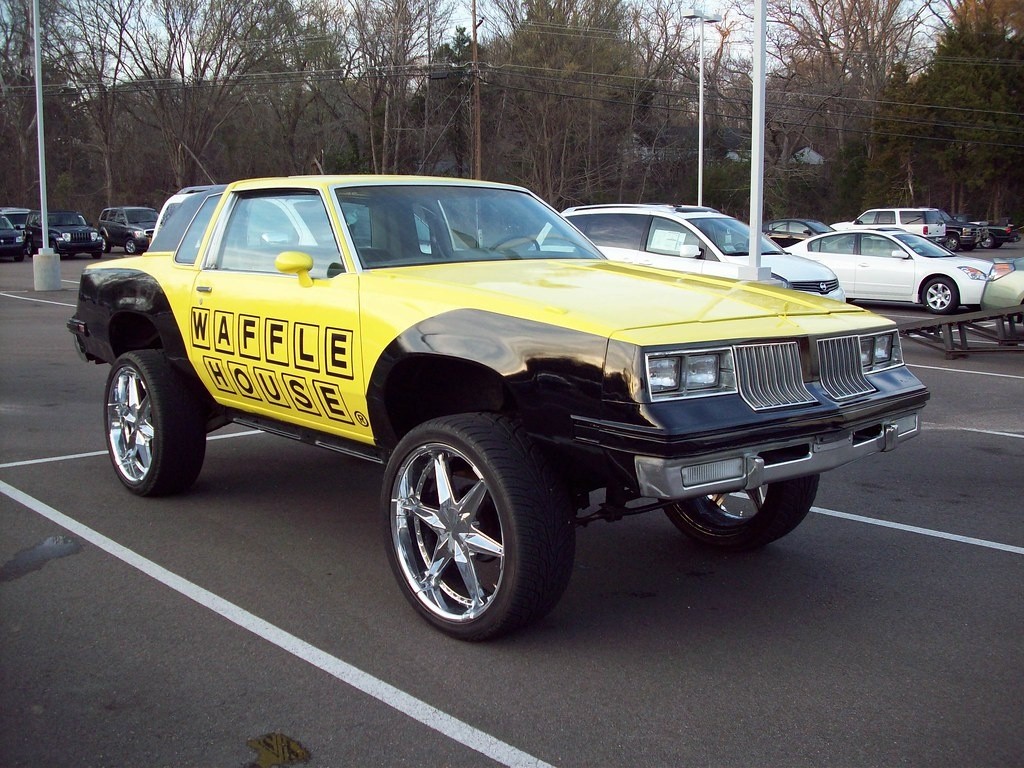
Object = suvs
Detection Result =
[528,202,847,304]
[149,185,448,259]
[24,209,103,259]
[0,215,25,262]
[64,173,933,645]
[829,207,1021,252]
[0,207,31,229]
[96,206,159,255]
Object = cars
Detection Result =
[781,227,993,315]
[980,257,1024,324]
[762,217,837,249]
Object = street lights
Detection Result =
[683,9,723,209]
